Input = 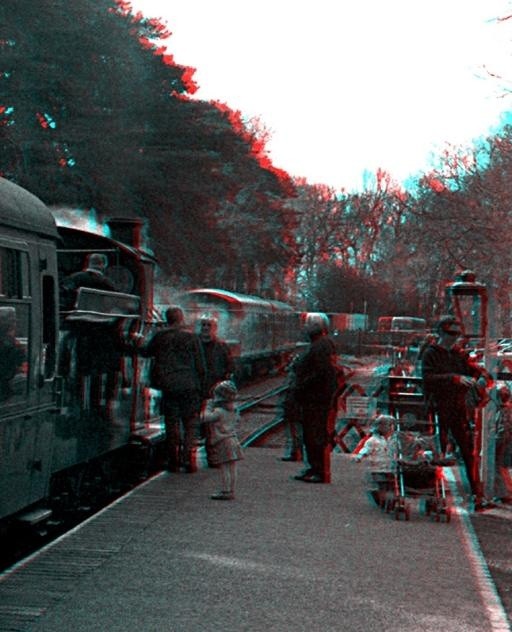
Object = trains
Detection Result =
[0,177,298,538]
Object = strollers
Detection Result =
[386,376,451,523]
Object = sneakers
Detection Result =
[163,462,198,473]
[210,490,235,500]
[474,500,498,511]
[213,380,237,402]
[294,469,330,485]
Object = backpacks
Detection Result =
[148,330,196,392]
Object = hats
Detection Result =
[439,315,463,333]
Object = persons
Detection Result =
[279,341,341,462]
[416,314,502,512]
[133,306,197,472]
[189,312,235,442]
[76,276,128,430]
[0,305,28,408]
[494,385,512,508]
[295,310,338,483]
[200,378,243,500]
[355,414,400,509]
[68,253,110,292]
